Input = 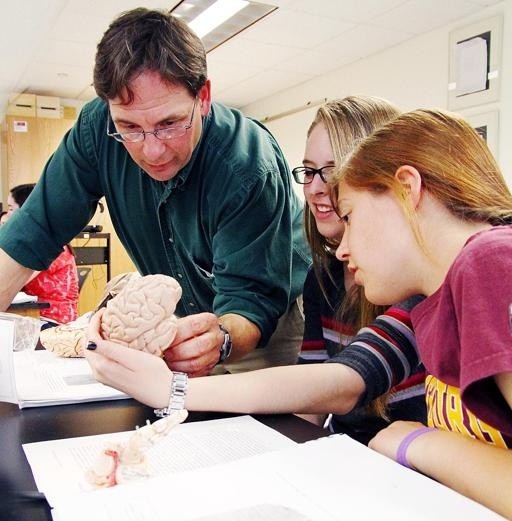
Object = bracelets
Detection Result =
[396,427,435,470]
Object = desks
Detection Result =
[0,321,512,521]
[6,301,50,319]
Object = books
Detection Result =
[13,350,132,409]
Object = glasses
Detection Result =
[293,165,337,184]
[107,97,196,144]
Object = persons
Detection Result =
[82,95,429,447]
[7,183,79,331]
[0,8,316,378]
[335,109,511,521]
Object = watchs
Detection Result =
[215,324,232,366]
[153,371,188,418]
